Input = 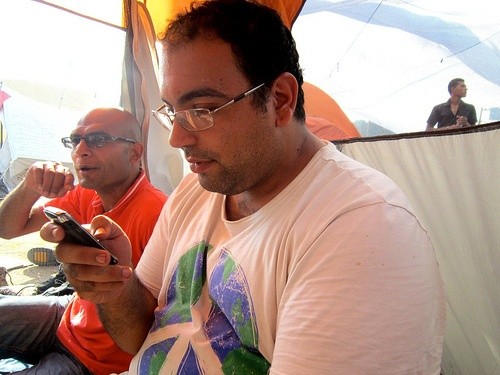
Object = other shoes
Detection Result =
[27,247,61,267]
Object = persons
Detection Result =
[0,107,173,375]
[426,78,477,131]
[27,247,60,266]
[41,1,446,374]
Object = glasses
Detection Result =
[60,134,137,152]
[151,82,266,133]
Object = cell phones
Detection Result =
[43,206,118,265]
